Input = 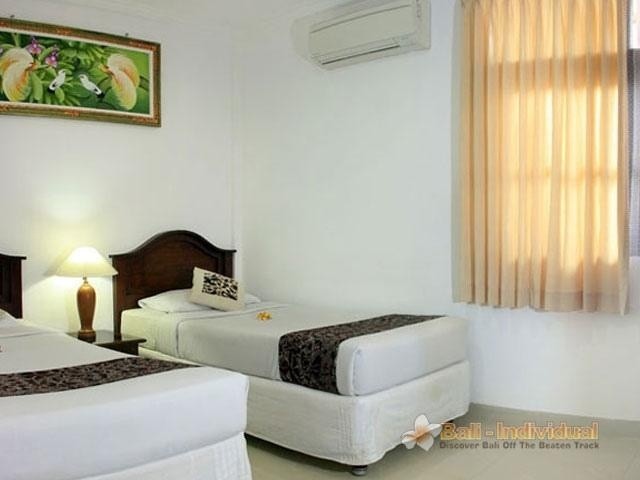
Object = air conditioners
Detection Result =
[290,1,431,71]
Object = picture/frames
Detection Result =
[0,16,162,128]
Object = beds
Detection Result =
[1,229,472,480]
